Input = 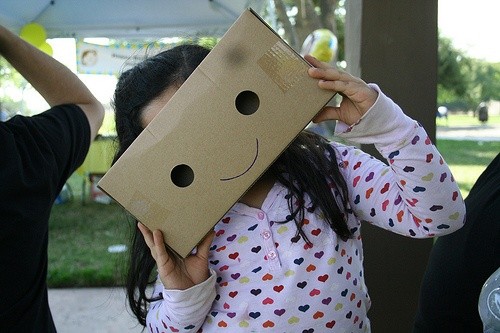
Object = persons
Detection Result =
[110,44,466,333]
[0,27,105,333]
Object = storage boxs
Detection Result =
[89,171,115,205]
[97,6,338,263]
